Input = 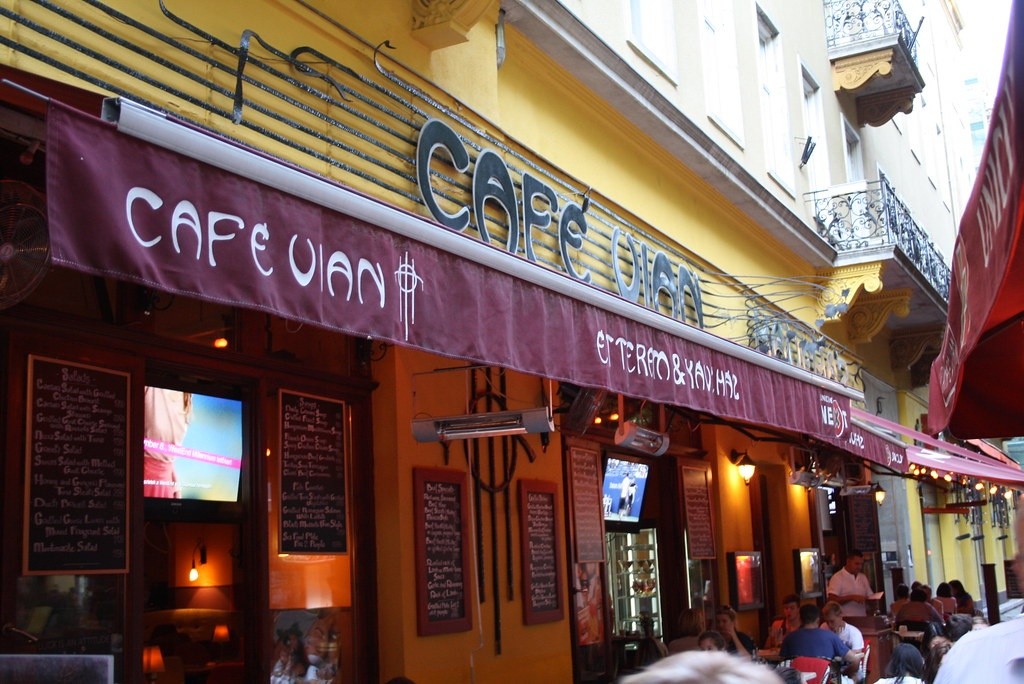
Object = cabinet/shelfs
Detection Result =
[604,518,664,653]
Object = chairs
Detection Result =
[900,620,930,655]
[173,641,211,674]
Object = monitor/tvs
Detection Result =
[136,378,252,523]
[603,457,650,533]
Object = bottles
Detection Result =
[776,628,783,653]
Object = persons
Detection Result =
[144,385,193,499]
[934,493,1024,684]
[635,637,662,667]
[697,631,724,651]
[619,473,636,517]
[668,609,704,654]
[819,600,865,684]
[779,604,860,676]
[871,580,975,684]
[715,604,754,659]
[270,610,335,684]
[827,550,875,616]
[763,594,802,648]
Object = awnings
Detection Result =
[0,64,866,438]
[814,407,1024,491]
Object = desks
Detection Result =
[757,649,784,663]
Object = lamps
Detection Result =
[868,480,888,508]
[142,640,168,684]
[995,534,1009,542]
[187,536,208,582]
[971,534,985,542]
[839,484,872,497]
[212,624,230,661]
[409,405,555,445]
[613,422,671,458]
[789,471,823,490]
[956,533,970,541]
[909,464,1013,499]
[732,448,758,486]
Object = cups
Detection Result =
[900,626,907,635]
[618,621,626,637]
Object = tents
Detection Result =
[922,0,1024,442]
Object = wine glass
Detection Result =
[618,559,655,573]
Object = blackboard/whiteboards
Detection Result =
[519,479,565,626]
[277,389,351,555]
[851,494,878,553]
[1004,560,1024,598]
[22,353,130,576]
[413,467,473,636]
[682,467,716,559]
[571,447,606,563]
